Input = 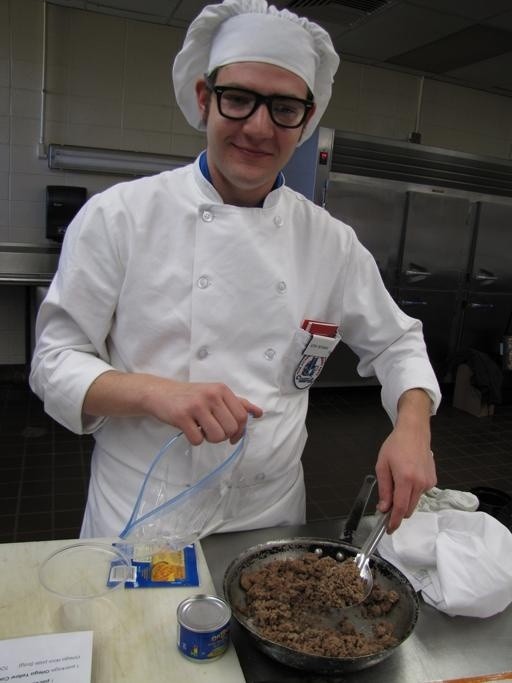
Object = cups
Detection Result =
[39,544,131,634]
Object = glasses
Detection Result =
[203,73,315,131]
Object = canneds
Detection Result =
[177,594,232,663]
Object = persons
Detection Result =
[24,0,441,538]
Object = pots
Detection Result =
[222,474,420,677]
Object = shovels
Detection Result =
[344,506,393,608]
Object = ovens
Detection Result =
[277,126,512,390]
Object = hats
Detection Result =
[172,1,341,149]
